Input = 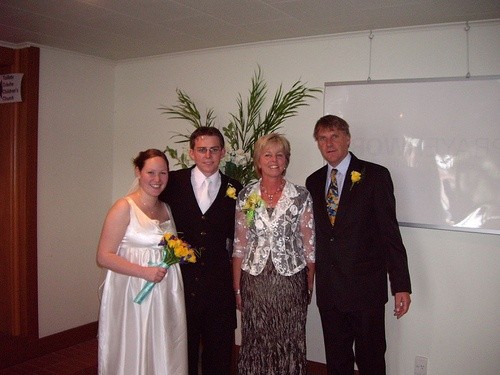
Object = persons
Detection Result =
[94,148,188,375]
[159,126,243,375]
[232,133,316,375]
[305,114,412,375]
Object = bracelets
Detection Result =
[232,289,242,295]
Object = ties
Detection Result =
[326,169,340,228]
[198,179,212,215]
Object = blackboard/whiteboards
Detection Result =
[323,75,500,234]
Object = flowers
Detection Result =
[133,233,197,302]
[349,169,362,192]
[240,194,262,227]
[224,186,238,200]
[220,145,251,178]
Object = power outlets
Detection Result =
[414,357,428,375]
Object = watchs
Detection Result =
[307,288,313,295]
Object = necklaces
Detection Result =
[137,190,159,215]
[261,179,284,206]
